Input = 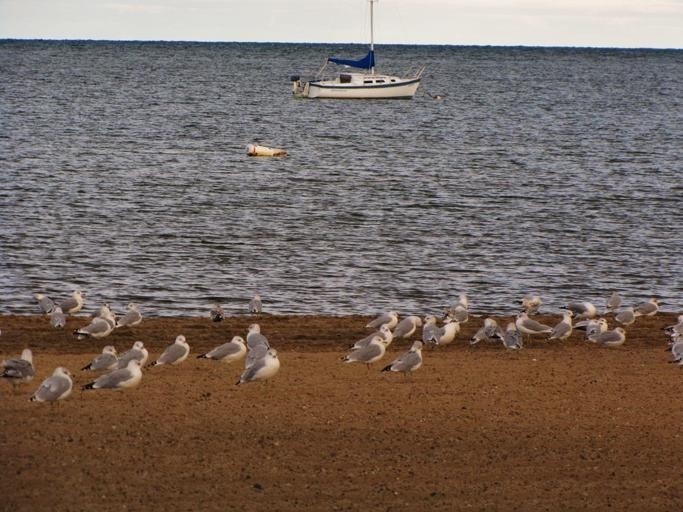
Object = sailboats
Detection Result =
[290,0,426,100]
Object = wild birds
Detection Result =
[3,290,280,407]
[340,290,683,378]
